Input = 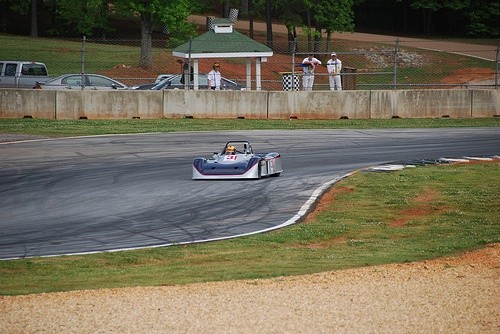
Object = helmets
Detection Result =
[226,145,236,155]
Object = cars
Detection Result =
[33,74,247,90]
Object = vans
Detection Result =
[0,61,48,88]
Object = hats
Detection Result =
[330,52,337,56]
[214,61,220,67]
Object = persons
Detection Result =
[326,52,342,91]
[301,54,322,90]
[226,146,236,155]
[207,62,223,90]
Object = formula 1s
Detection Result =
[192,140,283,179]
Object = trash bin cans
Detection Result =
[341,66,357,90]
